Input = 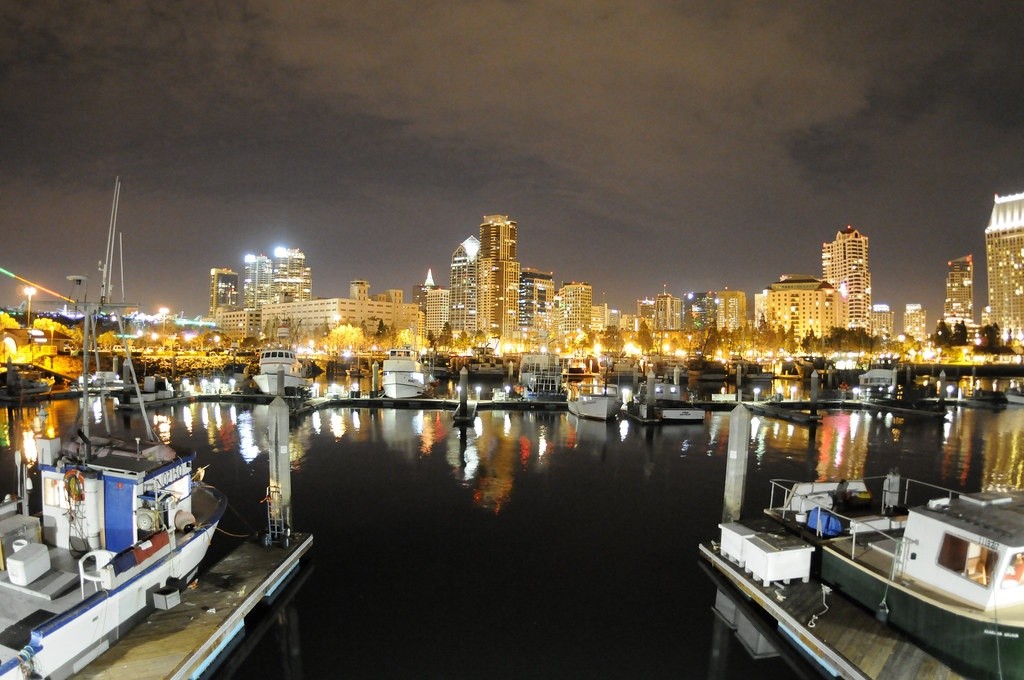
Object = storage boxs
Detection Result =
[6,543,51,586]
[152,587,181,611]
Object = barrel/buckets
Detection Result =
[12,538,29,553]
[806,506,841,536]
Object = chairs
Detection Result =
[78,549,119,600]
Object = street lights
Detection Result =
[160,307,169,348]
[21,288,38,328]
[137,331,158,376]
[185,336,194,360]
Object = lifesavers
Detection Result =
[63,469,85,501]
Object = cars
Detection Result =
[95,344,194,351]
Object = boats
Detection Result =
[345,367,368,376]
[517,354,624,421]
[70,371,124,392]
[632,376,707,424]
[0,275,228,679]
[856,369,895,401]
[382,343,437,399]
[1,364,57,398]
[252,342,314,396]
[426,357,506,382]
[765,466,1024,680]
